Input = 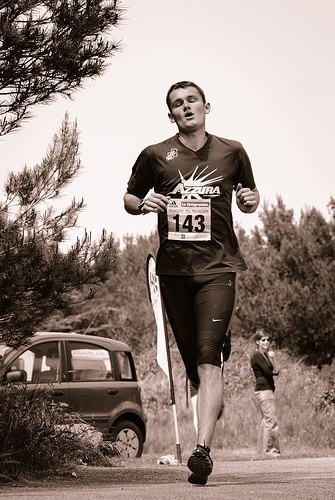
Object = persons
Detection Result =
[124,80,260,486]
[251,329,282,456]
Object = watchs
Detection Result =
[138,198,145,215]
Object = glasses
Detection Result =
[262,338,270,341]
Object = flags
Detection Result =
[145,253,169,384]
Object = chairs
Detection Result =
[73,369,112,379]
[36,348,58,383]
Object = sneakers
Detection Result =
[223,328,232,362]
[186,439,214,485]
[264,447,282,457]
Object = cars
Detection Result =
[0,331,147,461]
